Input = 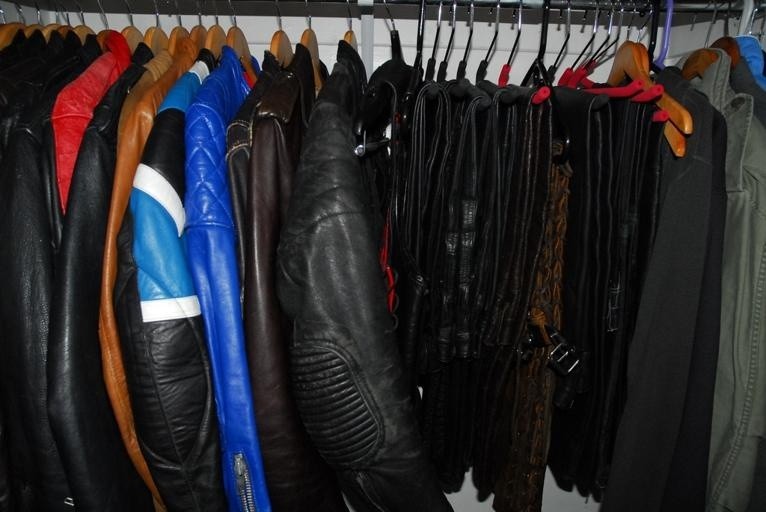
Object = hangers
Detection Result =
[0,0,766,158]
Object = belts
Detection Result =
[527,324,581,374]
